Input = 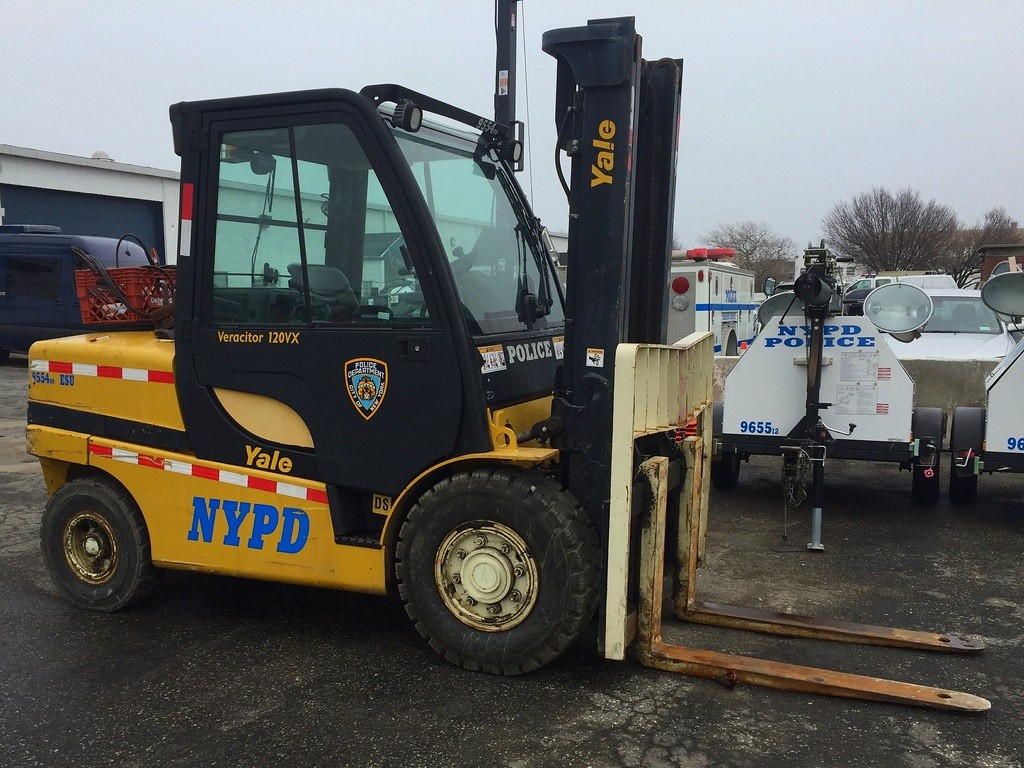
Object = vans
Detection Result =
[0,225,159,364]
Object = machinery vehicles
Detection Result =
[26,16,994,714]
[666,248,1024,507]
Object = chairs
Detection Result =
[952,303,980,330]
[287,264,395,322]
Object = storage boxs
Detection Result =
[74,267,177,326]
